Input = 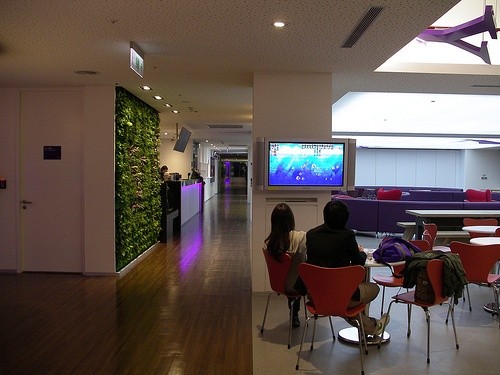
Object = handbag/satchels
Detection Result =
[414,268,434,305]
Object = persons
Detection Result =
[159,166,168,180]
[264,203,308,327]
[293,199,390,335]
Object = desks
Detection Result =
[338,248,406,345]
[406,210,500,240]
[470,237,500,314]
[462,226,500,236]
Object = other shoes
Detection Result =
[292,315,300,328]
[376,313,390,336]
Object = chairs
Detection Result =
[464,189,492,202]
[259,217,500,375]
[377,188,402,200]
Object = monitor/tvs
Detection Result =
[172,127,192,153]
[256,137,356,191]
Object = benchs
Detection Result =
[396,221,416,241]
[435,230,470,245]
[331,186,500,238]
